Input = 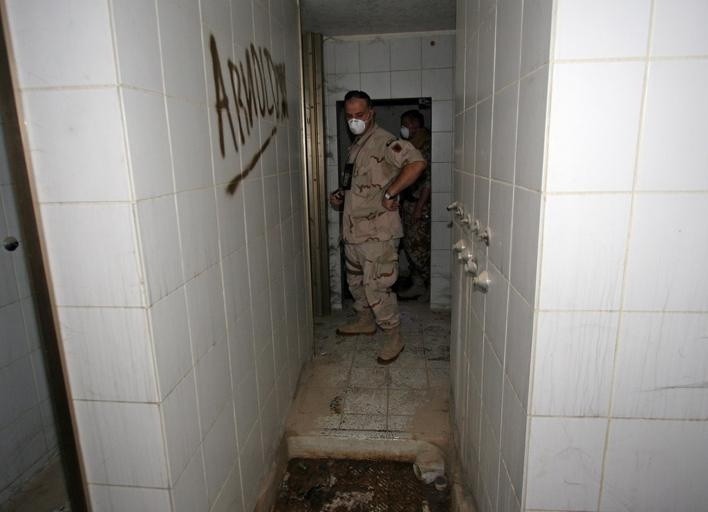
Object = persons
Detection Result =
[397,108,431,302]
[327,89,431,364]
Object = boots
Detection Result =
[398,273,427,299]
[337,307,377,335]
[378,320,405,364]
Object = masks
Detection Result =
[346,118,372,135]
[399,124,411,139]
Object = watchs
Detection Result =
[384,189,397,201]
